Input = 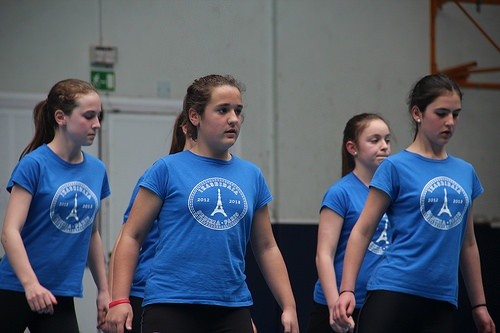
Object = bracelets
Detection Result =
[108,299,131,308]
[471,303,487,310]
[338,290,355,296]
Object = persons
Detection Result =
[310,113,392,332]
[0,79,112,332]
[332,72,497,333]
[103,75,299,333]
